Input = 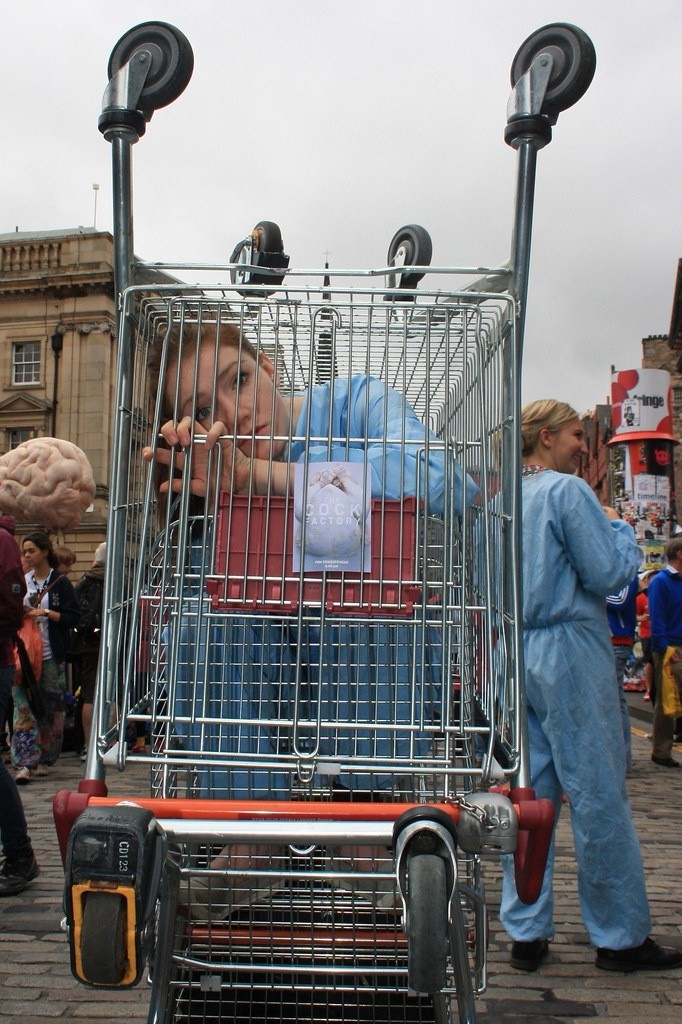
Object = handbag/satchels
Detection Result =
[14,611,43,684]
[662,647,682,716]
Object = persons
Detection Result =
[0,512,682,896]
[141,323,483,933]
[465,400,682,973]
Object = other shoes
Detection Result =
[509,941,550,972]
[645,732,655,742]
[127,746,146,756]
[325,844,411,916]
[0,859,40,896]
[35,765,50,776]
[643,690,650,700]
[14,768,30,784]
[651,754,679,767]
[596,937,681,972]
[173,849,287,923]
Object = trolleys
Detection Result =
[51,18,596,1022]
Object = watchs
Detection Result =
[45,609,50,616]
